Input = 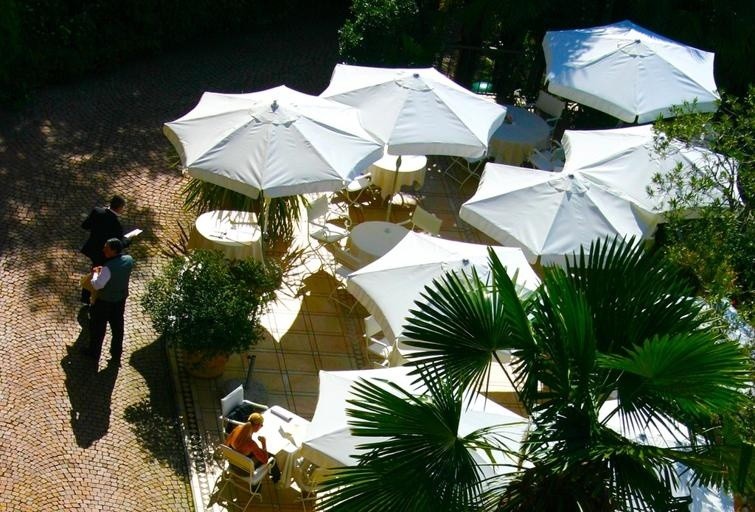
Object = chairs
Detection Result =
[304,194,351,278]
[532,90,566,138]
[443,154,488,191]
[213,443,278,511]
[360,313,395,369]
[323,246,366,318]
[408,205,443,237]
[290,463,326,512]
[215,382,268,444]
[344,172,375,208]
[527,139,569,172]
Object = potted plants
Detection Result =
[137,246,285,381]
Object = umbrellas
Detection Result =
[342,228,547,367]
[162,84,385,242]
[460,160,649,276]
[559,122,747,240]
[320,60,509,202]
[542,21,724,126]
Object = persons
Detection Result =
[86,237,134,366]
[81,194,133,282]
[223,412,281,493]
